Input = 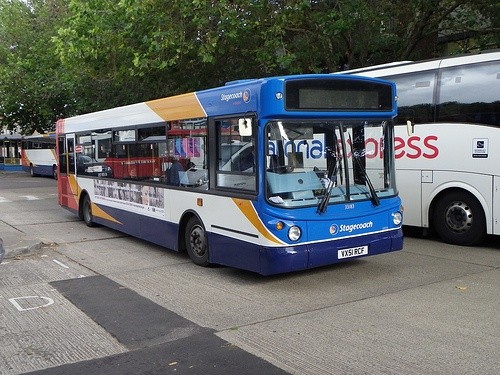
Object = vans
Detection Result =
[178,138,258,197]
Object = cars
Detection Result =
[61,151,114,179]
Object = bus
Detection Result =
[77,133,168,179]
[235,49,500,248]
[53,71,406,279]
[17,132,62,180]
